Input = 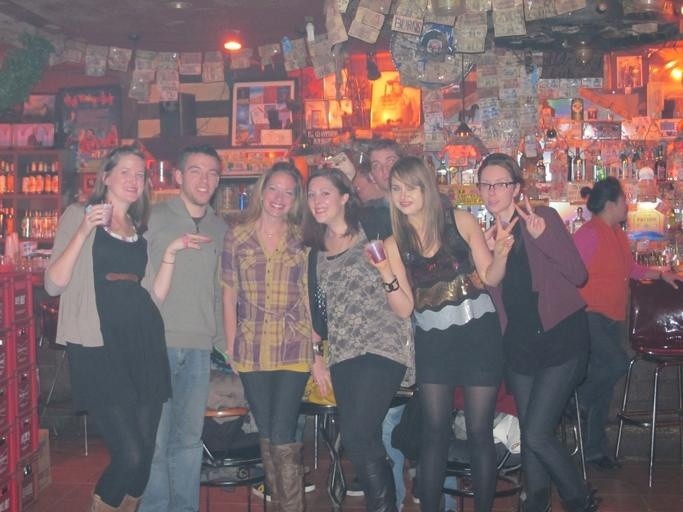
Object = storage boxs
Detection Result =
[36,425,54,495]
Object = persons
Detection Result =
[363,156,519,512]
[476,151,603,511]
[359,137,405,243]
[298,166,413,512]
[216,160,335,511]
[572,175,683,488]
[136,144,230,512]
[41,142,213,511]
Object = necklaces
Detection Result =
[267,233,273,239]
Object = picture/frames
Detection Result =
[226,73,300,151]
[609,43,650,97]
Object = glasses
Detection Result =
[476,182,515,191]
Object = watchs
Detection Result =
[312,340,324,357]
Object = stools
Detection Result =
[555,386,587,488]
[296,400,347,512]
[442,409,522,511]
[200,408,267,510]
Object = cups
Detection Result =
[92,199,112,228]
[365,239,386,264]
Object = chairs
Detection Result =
[611,276,683,489]
[31,295,93,458]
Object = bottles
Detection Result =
[0,207,58,238]
[552,143,668,182]
[0,253,48,274]
[221,187,232,209]
[466,204,496,234]
[239,187,248,210]
[572,207,587,235]
[1,160,61,195]
[635,248,679,269]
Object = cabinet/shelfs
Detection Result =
[0,145,73,284]
[215,169,264,214]
[0,270,43,512]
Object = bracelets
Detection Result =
[161,260,176,265]
[382,277,400,293]
[659,270,662,280]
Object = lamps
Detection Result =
[453,44,472,137]
[364,43,381,83]
